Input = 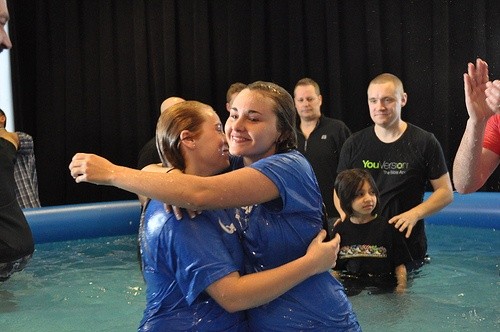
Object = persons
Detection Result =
[0,108,42,208]
[0,128,35,281]
[336,73,454,277]
[68,78,361,332]
[327,168,410,296]
[452,58,500,193]
